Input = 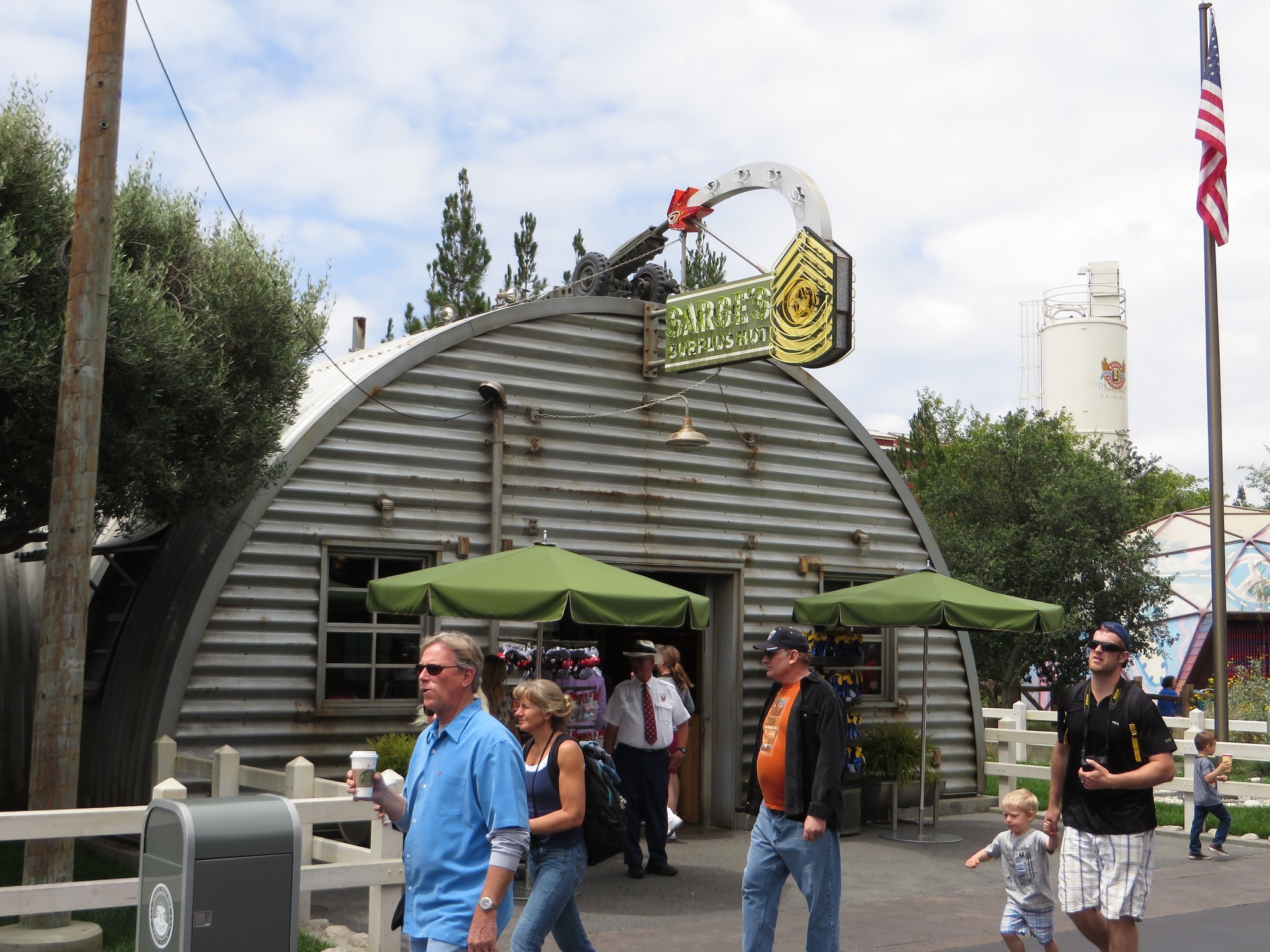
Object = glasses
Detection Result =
[1087,639,1125,652]
[413,663,460,675]
[629,657,651,664]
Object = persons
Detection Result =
[1190,8,1232,252]
[478,654,521,743]
[630,644,696,844]
[345,630,531,952]
[601,640,692,880]
[1187,731,1232,859]
[738,626,848,951]
[964,788,1061,952]
[1042,621,1178,952]
[1157,676,1180,717]
[510,678,599,952]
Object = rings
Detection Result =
[1082,780,1086,785]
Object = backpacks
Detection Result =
[523,734,630,866]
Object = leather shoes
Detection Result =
[627,863,678,878]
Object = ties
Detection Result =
[641,682,657,746]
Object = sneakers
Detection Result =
[1188,850,1211,860]
[1208,842,1230,857]
[666,814,684,842]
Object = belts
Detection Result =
[616,741,667,754]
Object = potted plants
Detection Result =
[845,716,947,810]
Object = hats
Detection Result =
[623,640,663,664]
[1093,621,1130,668]
[752,625,811,654]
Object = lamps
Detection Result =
[641,393,710,453]
[375,493,396,528]
[852,529,870,557]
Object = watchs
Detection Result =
[677,747,687,754]
[478,896,500,912]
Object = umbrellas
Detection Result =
[795,557,1069,845]
[363,528,713,680]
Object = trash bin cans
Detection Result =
[133,792,302,952]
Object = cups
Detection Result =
[1222,753,1233,772]
[379,777,398,824]
[350,751,379,797]
